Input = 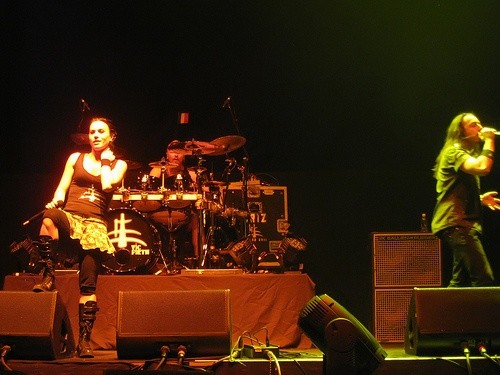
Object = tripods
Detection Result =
[202,186,230,270]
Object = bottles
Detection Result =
[420,213,428,233]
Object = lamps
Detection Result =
[297,294,388,375]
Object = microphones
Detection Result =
[81,99,90,111]
[222,96,231,108]
[494,131,500,136]
[23,200,64,226]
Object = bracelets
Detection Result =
[101,159,111,167]
[480,149,496,160]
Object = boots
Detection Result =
[76,300,99,358]
[32,235,59,293]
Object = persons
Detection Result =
[431,113,500,288]
[134,140,207,268]
[31,117,127,357]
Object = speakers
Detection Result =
[370,231,500,357]
[116,288,233,360]
[0,291,76,360]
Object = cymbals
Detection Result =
[206,135,247,156]
[167,141,218,156]
[148,161,180,169]
[202,180,231,186]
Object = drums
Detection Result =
[108,189,129,207]
[151,206,189,226]
[166,190,191,209]
[100,207,162,274]
[131,192,162,212]
